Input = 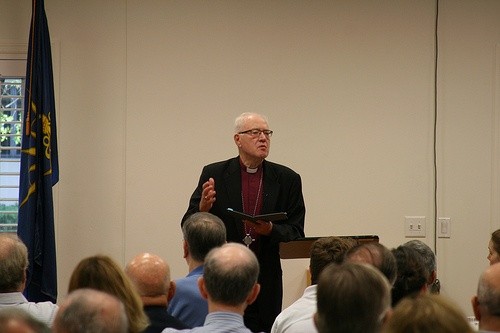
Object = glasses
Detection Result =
[238,129,273,137]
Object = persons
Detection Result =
[0,212,500,333]
[181,111,306,266]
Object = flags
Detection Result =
[17,0,60,304]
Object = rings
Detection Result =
[204,196,208,200]
